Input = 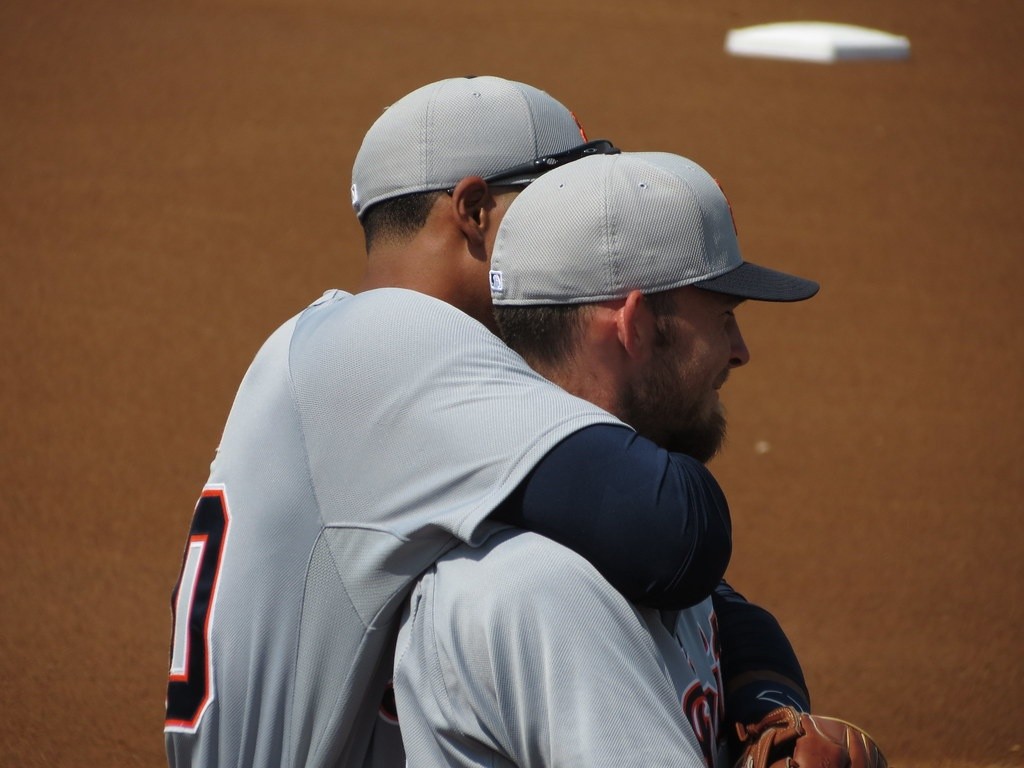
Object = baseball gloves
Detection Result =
[726,705,888,768]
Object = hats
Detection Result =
[489,149,821,306]
[351,75,589,218]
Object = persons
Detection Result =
[164,76,732,768]
[392,148,891,767]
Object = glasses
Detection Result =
[446,140,614,198]
